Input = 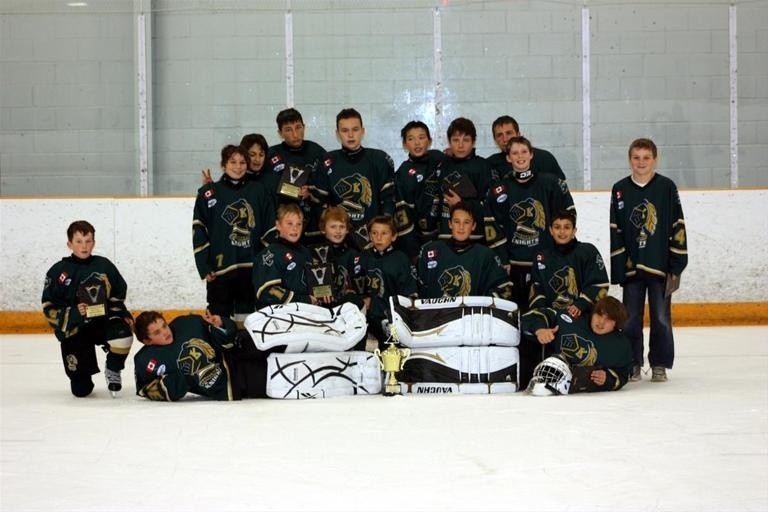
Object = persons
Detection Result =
[133,107,635,402]
[41,220,135,397]
[609,138,688,382]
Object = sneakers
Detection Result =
[105,366,122,391]
[630,365,642,381]
[653,368,669,381]
